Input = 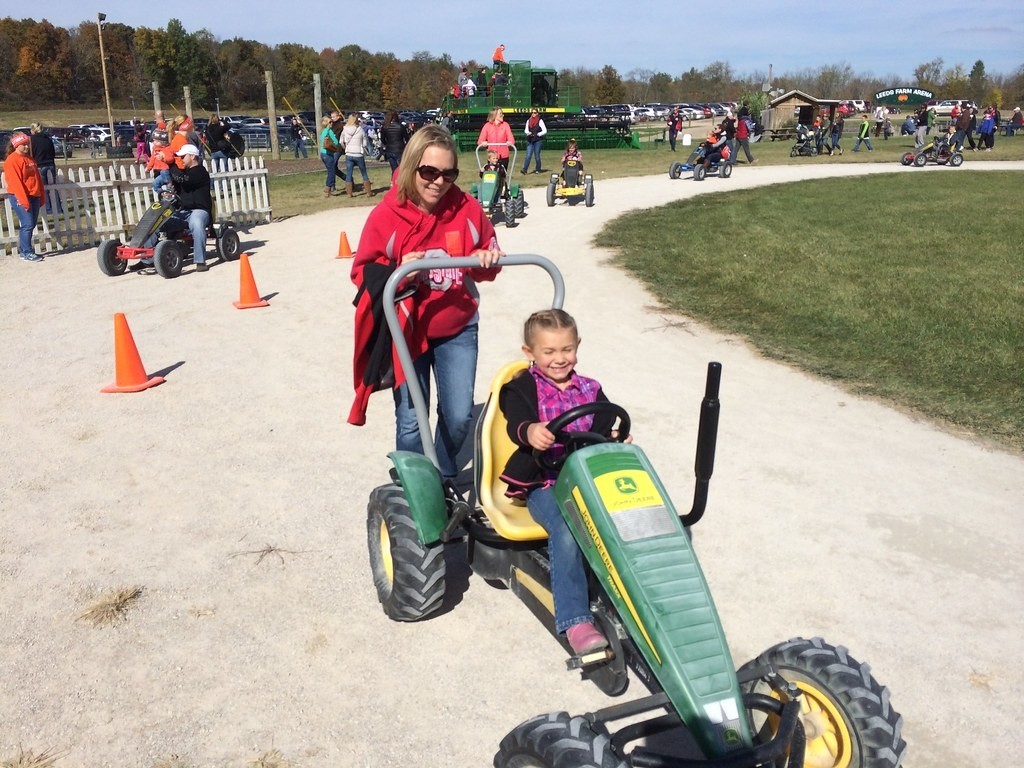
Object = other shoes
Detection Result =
[818,147,875,156]
[521,168,527,175]
[197,262,209,272]
[129,261,155,272]
[750,159,759,164]
[135,160,138,163]
[331,190,340,196]
[958,145,994,152]
[323,187,330,197]
[535,170,540,174]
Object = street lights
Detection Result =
[97,13,115,148]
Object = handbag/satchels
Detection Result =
[721,146,731,158]
[324,131,337,152]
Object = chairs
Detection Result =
[182,198,217,239]
[472,359,549,540]
[711,140,735,165]
[951,142,957,151]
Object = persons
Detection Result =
[694,108,759,166]
[320,110,414,197]
[291,119,308,159]
[934,103,1001,159]
[4,131,46,262]
[493,45,506,73]
[874,104,937,149]
[452,68,512,109]
[133,110,195,196]
[667,107,681,151]
[443,112,456,134]
[852,115,874,152]
[814,112,845,155]
[520,109,547,175]
[1006,107,1024,136]
[479,150,507,207]
[498,309,633,656]
[129,144,212,271]
[350,125,506,480]
[477,109,515,168]
[560,140,584,189]
[90,132,102,157]
[31,122,63,214]
[207,114,230,190]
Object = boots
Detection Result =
[364,181,376,197]
[346,182,356,197]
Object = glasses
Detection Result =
[988,108,993,109]
[20,143,29,147]
[181,154,187,159]
[328,121,334,123]
[715,127,720,130]
[415,165,460,183]
[532,112,538,114]
[921,107,926,109]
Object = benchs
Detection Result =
[872,126,885,134]
[997,120,1024,136]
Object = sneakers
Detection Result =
[19,251,43,262]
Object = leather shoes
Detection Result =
[565,622,609,658]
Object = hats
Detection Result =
[1014,107,1021,111]
[176,143,200,156]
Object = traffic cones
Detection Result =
[101,313,165,393]
[335,232,355,259]
[233,253,269,309]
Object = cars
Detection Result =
[925,100,979,116]
[0,108,441,160]
[819,100,902,118]
[575,102,740,125]
